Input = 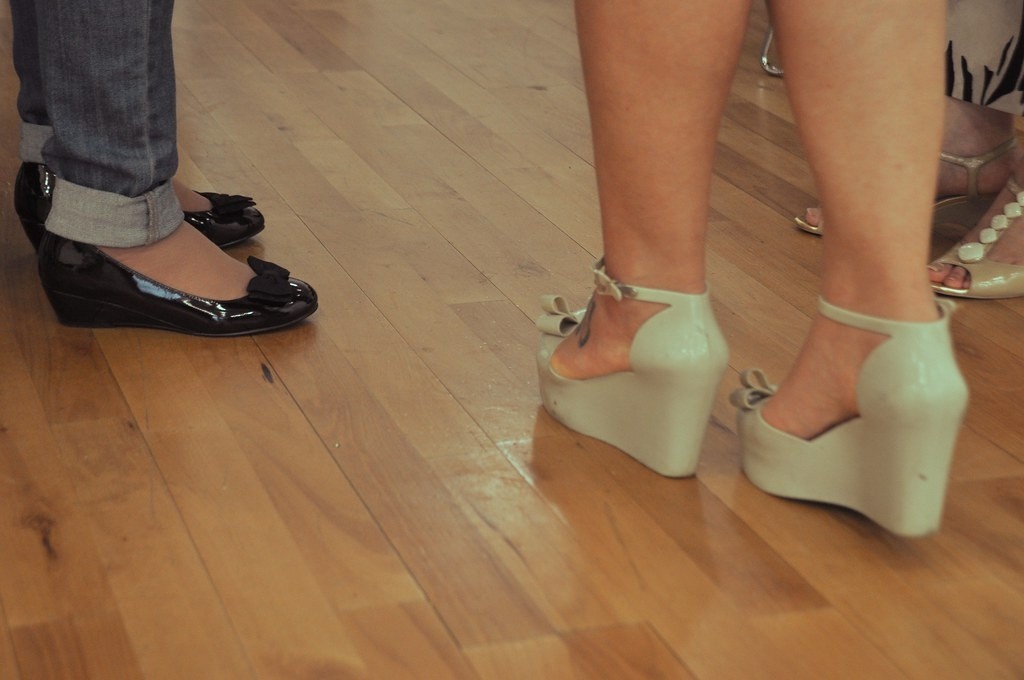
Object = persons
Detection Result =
[537,0,967,538]
[795,0,1024,300]
[9,0,318,338]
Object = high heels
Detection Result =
[732,296,970,536]
[539,256,731,478]
[794,138,1017,234]
[15,162,266,250]
[931,176,1024,299]
[36,231,319,336]
